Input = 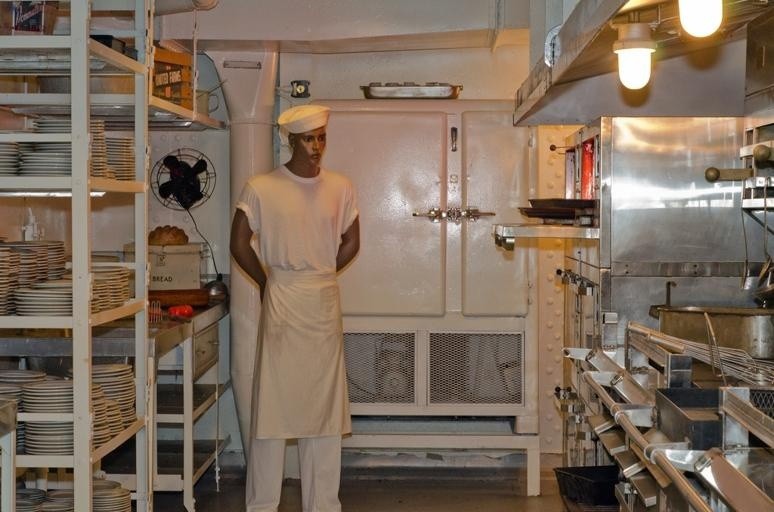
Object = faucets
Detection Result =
[665,279,676,307]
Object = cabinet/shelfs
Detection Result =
[0,0,156,510]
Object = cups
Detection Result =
[197,90,219,116]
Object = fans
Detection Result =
[149,147,217,282]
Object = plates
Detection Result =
[0,241,130,317]
[16,478,134,512]
[1,364,138,458]
[1,113,136,180]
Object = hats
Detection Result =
[277,104,332,135]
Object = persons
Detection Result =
[231,106,360,512]
